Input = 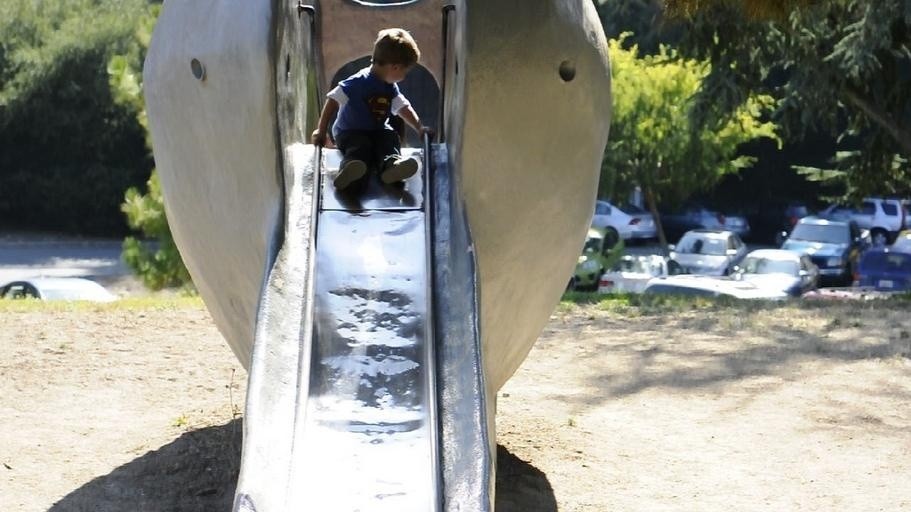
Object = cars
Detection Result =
[1,276,113,302]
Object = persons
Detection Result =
[311,27,435,190]
[715,212,726,231]
[850,243,862,280]
[392,114,405,145]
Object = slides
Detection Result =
[233,0,493,512]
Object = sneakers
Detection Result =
[381,157,418,185]
[334,160,367,190]
